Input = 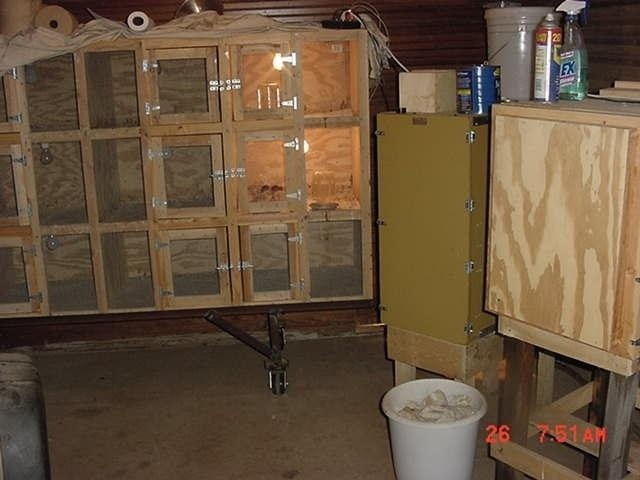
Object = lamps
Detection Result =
[46,232,58,250]
[40,143,53,164]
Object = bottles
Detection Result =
[456,71,472,114]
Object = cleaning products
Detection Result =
[554,0,589,101]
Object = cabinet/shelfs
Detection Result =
[486,99,639,376]
[0,29,374,320]
[376,112,504,390]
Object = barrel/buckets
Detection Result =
[484,7,555,102]
